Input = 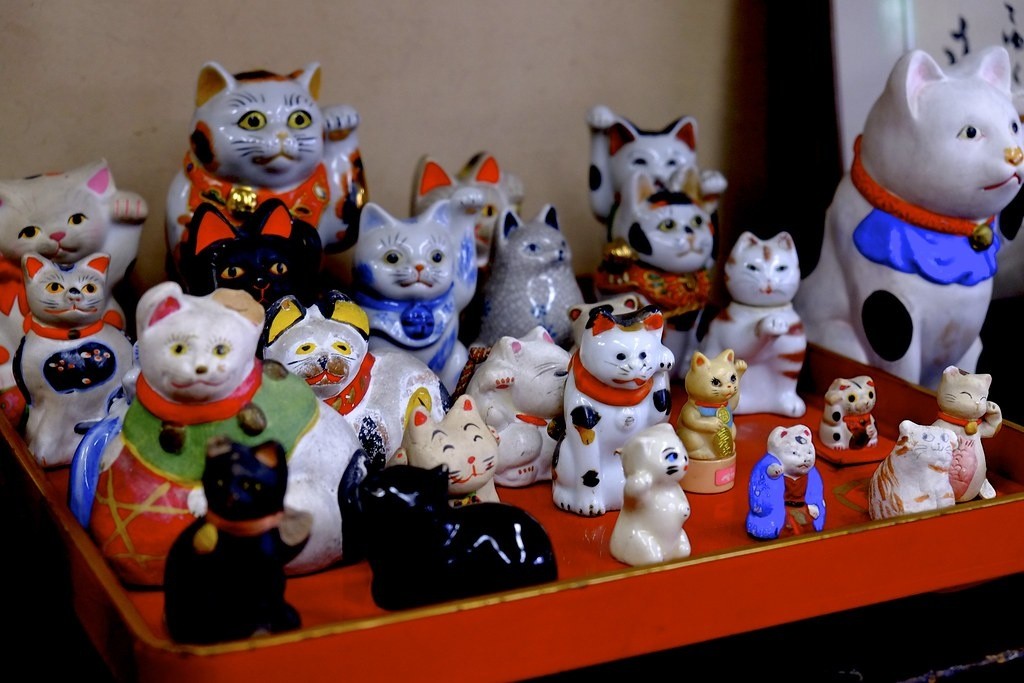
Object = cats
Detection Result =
[679,349,1006,537]
[588,107,728,380]
[551,290,693,566]
[701,44,1024,417]
[88,53,585,609]
[158,434,313,638]
[0,156,149,468]
